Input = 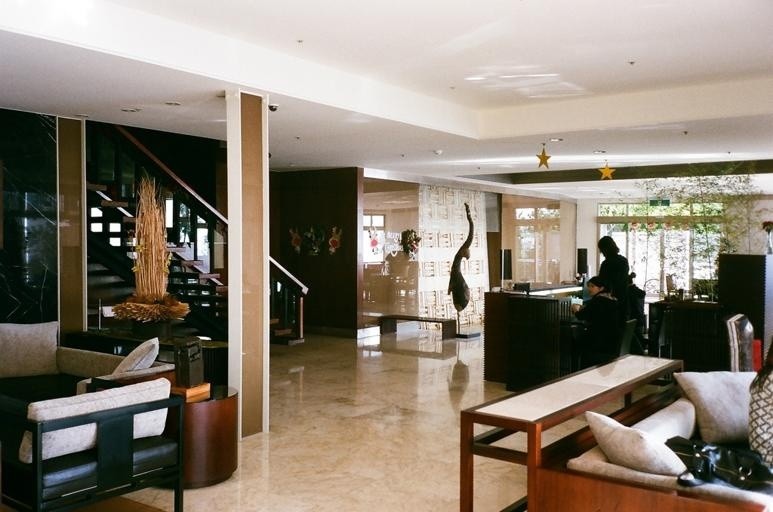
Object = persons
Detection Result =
[567,278,619,373]
[597,234,633,364]
[627,269,646,357]
[745,336,772,471]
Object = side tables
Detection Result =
[107,373,238,488]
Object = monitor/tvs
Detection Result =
[514,282,530,294]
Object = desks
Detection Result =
[460,354,683,512]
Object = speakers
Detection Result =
[577,248,587,275]
[503,249,512,280]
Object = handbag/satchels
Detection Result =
[665,435,769,491]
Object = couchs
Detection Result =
[535,389,767,512]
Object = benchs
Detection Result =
[378,315,456,341]
[0,380,185,512]
[0,323,176,416]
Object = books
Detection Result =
[169,380,212,398]
[184,392,210,403]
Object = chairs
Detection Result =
[726,314,753,372]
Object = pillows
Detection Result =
[585,410,686,477]
[672,371,758,443]
[111,337,159,375]
[19,375,172,463]
